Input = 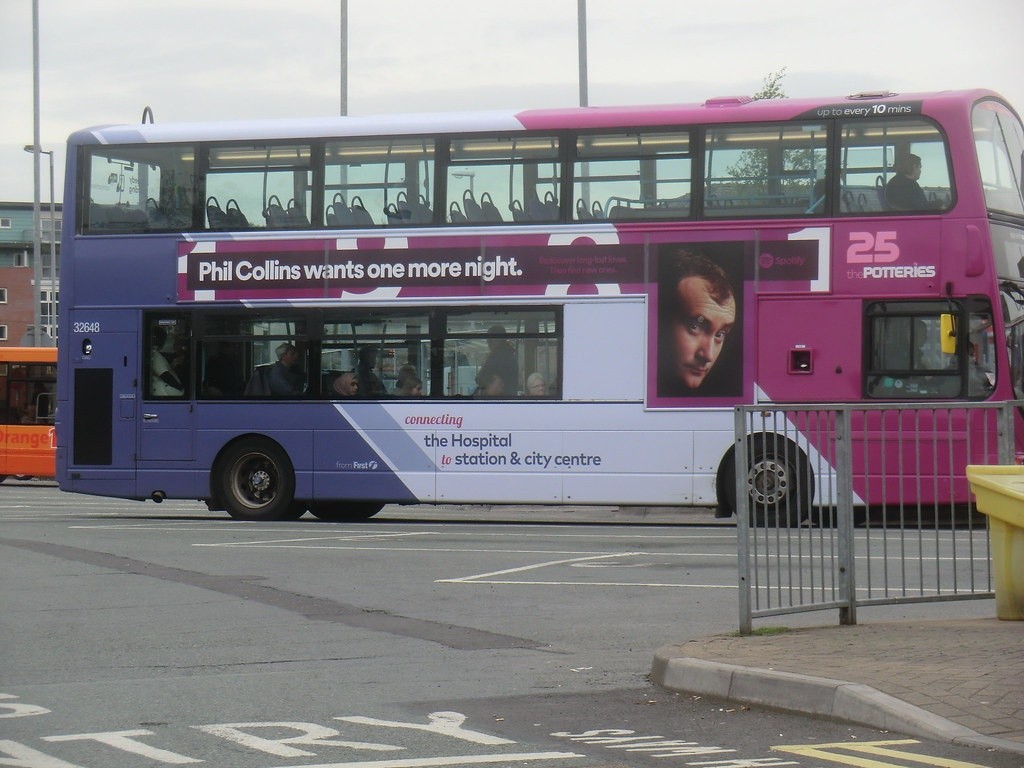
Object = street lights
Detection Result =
[24,143,57,348]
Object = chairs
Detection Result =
[245,367,273,398]
[141,176,946,225]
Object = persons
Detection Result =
[268,343,305,397]
[886,154,929,210]
[944,340,994,394]
[905,316,928,370]
[473,324,519,397]
[528,374,546,393]
[391,365,424,396]
[331,345,387,398]
[143,325,188,397]
[661,250,735,389]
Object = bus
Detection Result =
[54,87,1024,528]
[0,347,59,482]
